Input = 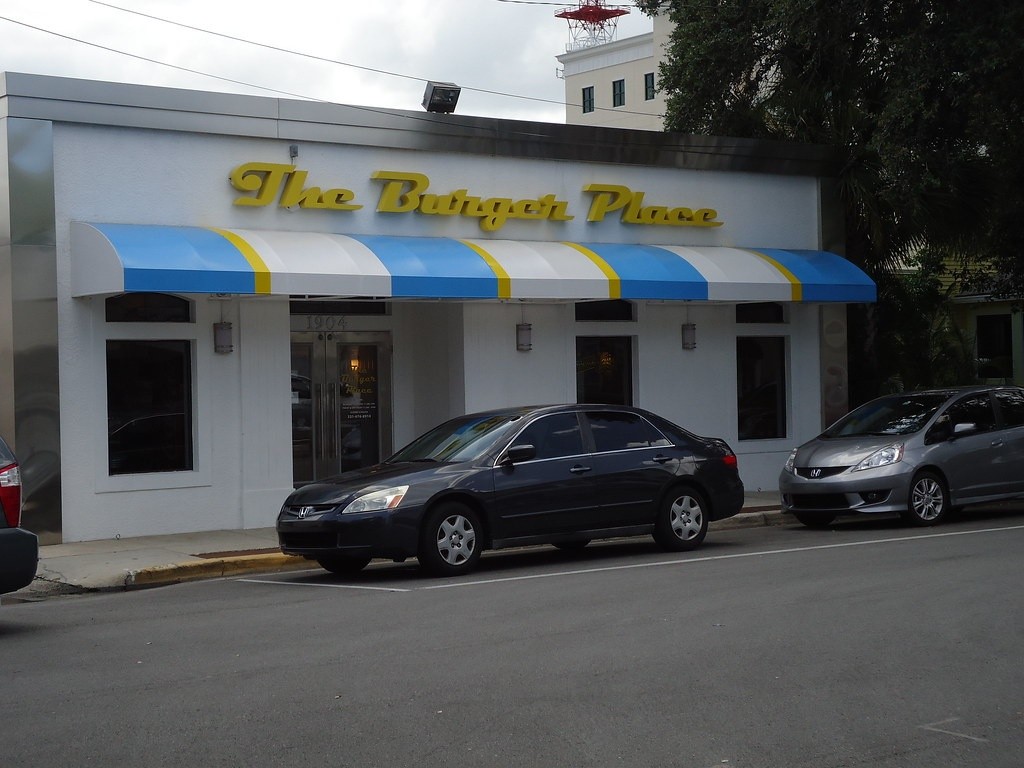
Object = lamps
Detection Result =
[421,81,462,114]
[515,323,532,351]
[682,323,697,349]
[213,322,234,353]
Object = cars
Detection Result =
[289,373,380,471]
[20,404,192,536]
[274,402,746,581]
[777,383,1024,531]
